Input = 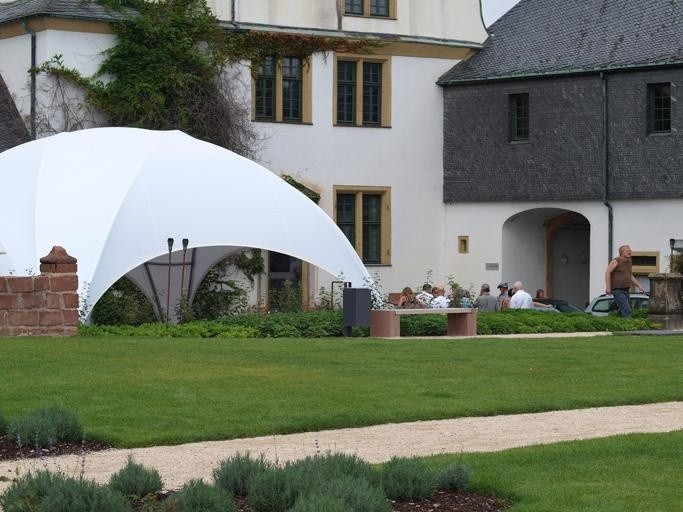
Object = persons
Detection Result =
[397,280,553,312]
[605,244,646,319]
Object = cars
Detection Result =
[531,298,587,315]
[584,291,650,315]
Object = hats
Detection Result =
[497,284,508,288]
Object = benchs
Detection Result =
[370,306,477,338]
